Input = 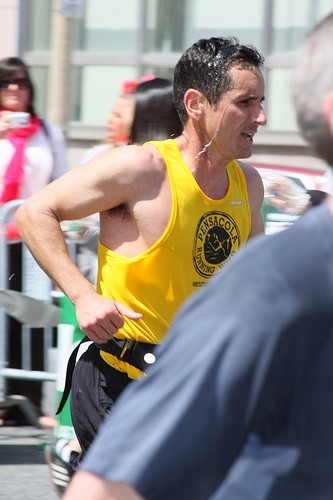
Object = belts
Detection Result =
[55,335,160,416]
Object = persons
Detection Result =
[46,79,184,496]
[62,11,332,496]
[15,40,268,459]
[1,58,68,427]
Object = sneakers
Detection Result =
[45,444,73,494]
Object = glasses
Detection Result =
[0,78,29,88]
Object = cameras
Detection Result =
[1,112,30,129]
